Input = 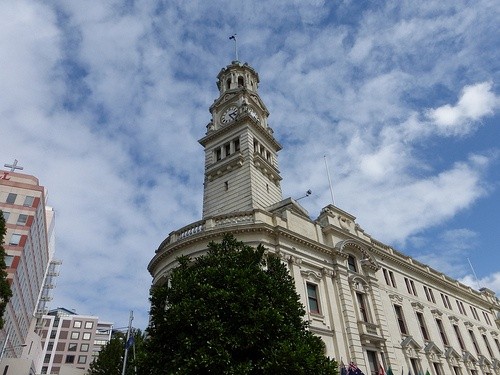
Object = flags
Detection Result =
[341,361,347,375]
[388,368,394,375]
[230,35,235,41]
[419,369,422,375]
[349,361,364,375]
[380,366,385,375]
[409,369,411,374]
[427,370,430,375]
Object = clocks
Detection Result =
[248,104,261,123]
[219,103,240,127]
